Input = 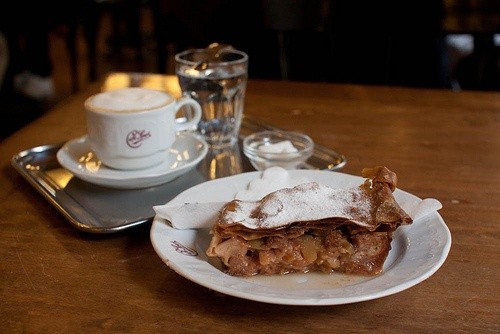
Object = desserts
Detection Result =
[206,166,414,277]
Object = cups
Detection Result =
[83,88,203,172]
[176,49,249,150]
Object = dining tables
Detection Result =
[0,70,500,334]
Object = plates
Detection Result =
[149,169,454,307]
[58,133,208,191]
[242,131,314,168]
